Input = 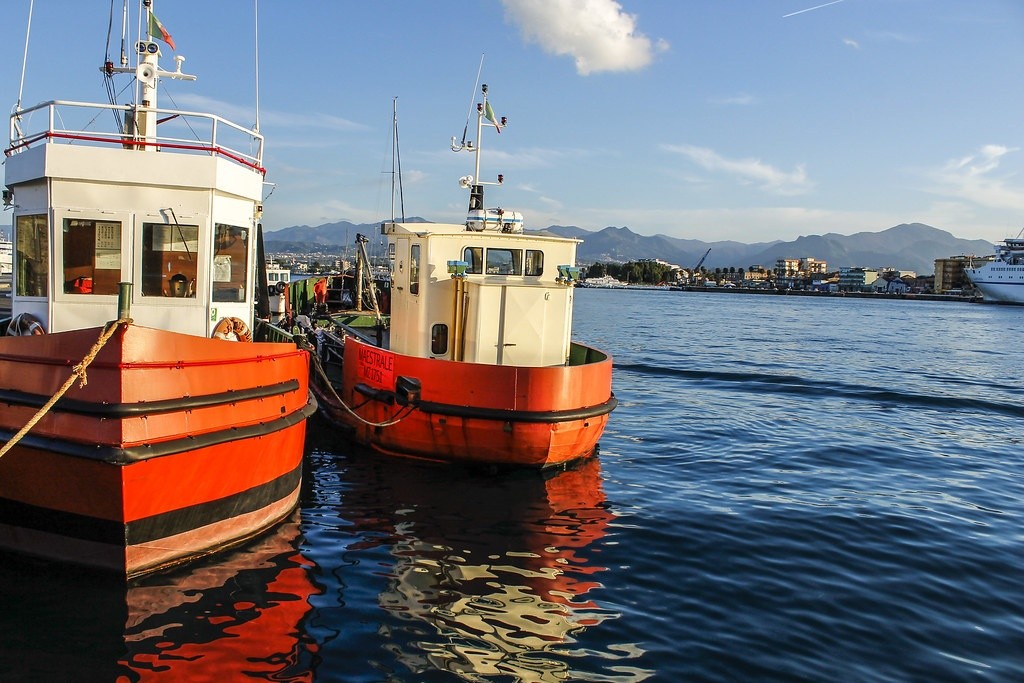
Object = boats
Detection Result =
[0,0,319,582]
[264,250,291,314]
[255,55,618,473]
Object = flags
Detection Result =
[148,13,175,50]
[484,102,501,133]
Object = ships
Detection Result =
[963,236,1024,305]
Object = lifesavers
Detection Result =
[212,317,253,343]
[7,313,46,336]
